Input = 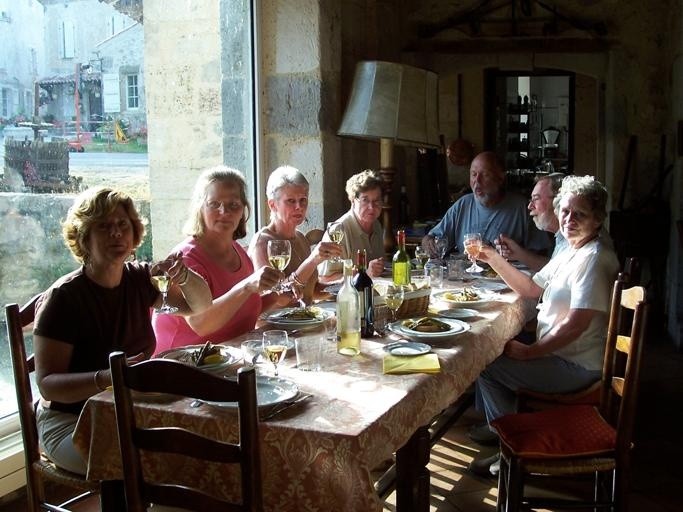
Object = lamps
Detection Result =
[334,59,440,259]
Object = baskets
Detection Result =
[373,281,431,318]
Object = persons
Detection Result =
[493,171,616,273]
[463,176,622,479]
[31,186,213,476]
[317,170,387,284]
[421,152,555,259]
[246,165,343,329]
[149,166,305,361]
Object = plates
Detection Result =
[198,375,298,412]
[470,269,532,281]
[438,308,478,320]
[472,282,507,291]
[260,308,337,325]
[433,288,496,307]
[399,320,463,335]
[388,318,470,337]
[382,342,433,355]
[153,344,243,373]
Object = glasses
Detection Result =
[205,198,243,211]
[528,194,554,205]
[355,195,385,206]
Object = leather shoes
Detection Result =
[468,452,501,477]
[468,423,500,447]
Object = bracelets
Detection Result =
[177,266,189,287]
[93,369,103,393]
[290,271,306,289]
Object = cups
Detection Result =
[385,284,404,324]
[267,240,292,291]
[241,340,268,375]
[463,233,483,272]
[262,330,287,377]
[327,222,346,264]
[148,260,178,314]
[434,236,446,263]
[424,266,442,289]
[321,311,338,341]
[295,337,323,372]
[447,260,460,280]
[411,276,429,291]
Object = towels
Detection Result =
[383,353,440,374]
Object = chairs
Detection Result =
[3,291,125,511]
[511,251,636,413]
[487,272,647,512]
[109,350,264,512]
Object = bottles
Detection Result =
[352,248,374,339]
[336,259,361,357]
[392,230,412,285]
